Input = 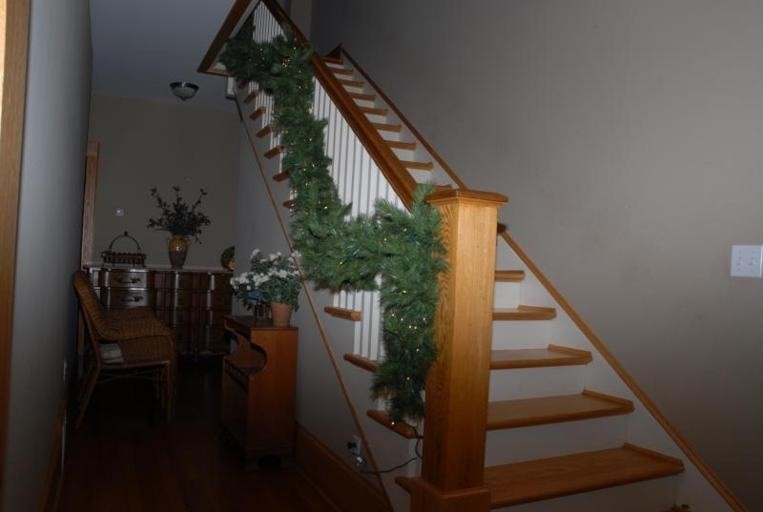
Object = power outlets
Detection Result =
[345,435,362,456]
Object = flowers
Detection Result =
[229,247,305,317]
[145,183,212,244]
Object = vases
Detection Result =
[168,234,188,269]
[270,301,293,328]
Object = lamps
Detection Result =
[170,82,199,101]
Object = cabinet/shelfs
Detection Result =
[99,267,234,368]
[219,315,299,467]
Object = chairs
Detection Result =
[66,270,177,433]
